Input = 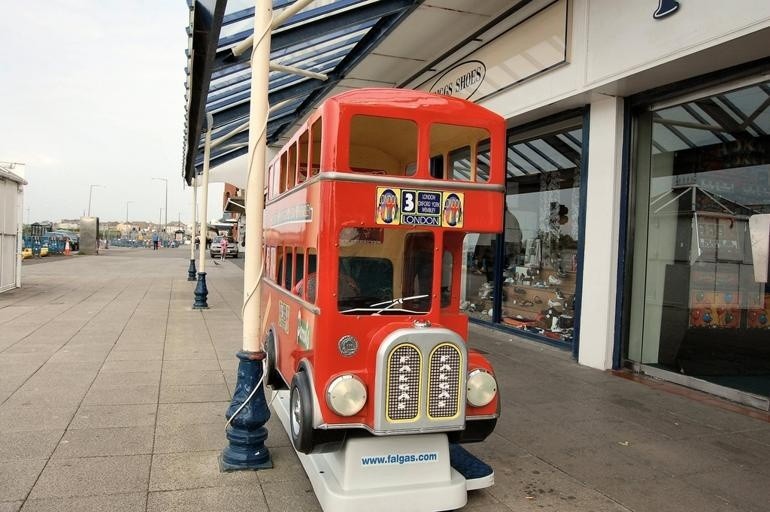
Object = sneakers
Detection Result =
[459,262,574,339]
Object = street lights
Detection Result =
[151,176,167,232]
[88,184,106,216]
[126,201,133,222]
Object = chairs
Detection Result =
[292,271,364,304]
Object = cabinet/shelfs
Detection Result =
[483,247,578,329]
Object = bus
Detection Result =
[262,88,507,453]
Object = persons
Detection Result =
[206,236,213,249]
[472,202,523,300]
[220,236,229,261]
[195,237,200,249]
[152,231,159,250]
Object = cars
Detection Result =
[22,230,78,259]
[209,236,238,258]
